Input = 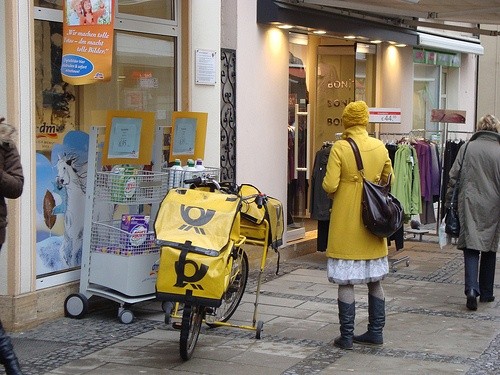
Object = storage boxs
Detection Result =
[238,201,286,251]
[155,243,235,307]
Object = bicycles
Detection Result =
[153,173,284,357]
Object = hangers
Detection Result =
[383,131,430,146]
[447,131,472,142]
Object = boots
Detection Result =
[353,293,386,346]
[0,329,24,375]
[333,298,356,349]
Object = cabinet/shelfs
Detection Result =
[65,124,222,324]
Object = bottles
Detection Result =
[169,158,206,189]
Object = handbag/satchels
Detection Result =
[444,199,461,238]
[362,179,404,238]
[439,213,447,249]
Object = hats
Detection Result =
[341,100,369,127]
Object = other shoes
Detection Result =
[466,289,477,310]
[480,295,495,302]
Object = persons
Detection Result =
[322,100,394,350]
[444,114,500,311]
[68,0,105,25]
[0,122,24,375]
